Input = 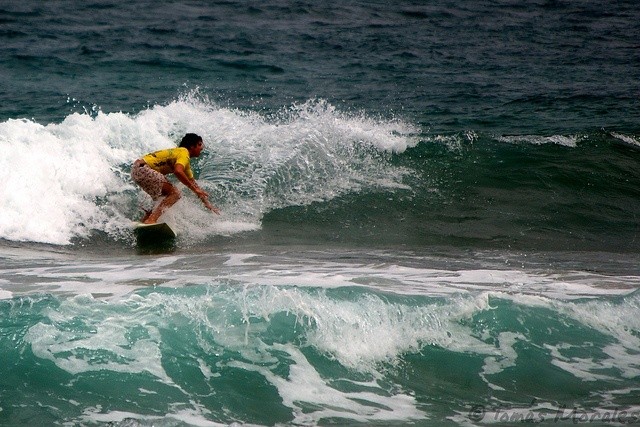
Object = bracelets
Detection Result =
[191,183,198,190]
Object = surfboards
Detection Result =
[134,209,175,248]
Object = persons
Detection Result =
[131,133,221,224]
